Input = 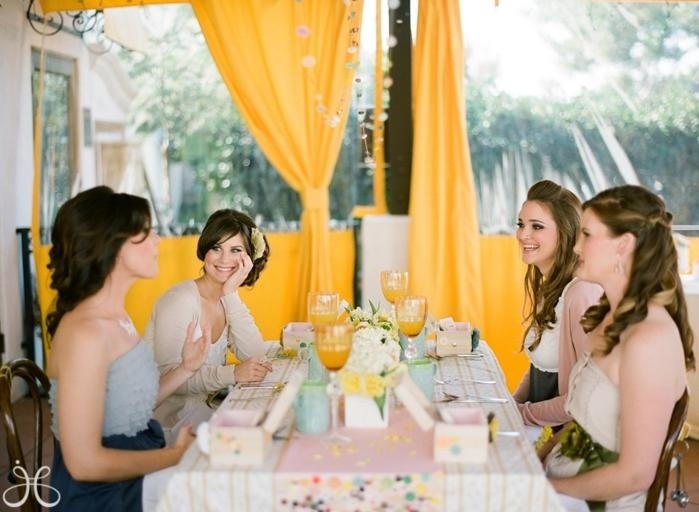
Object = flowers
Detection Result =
[346,299,400,368]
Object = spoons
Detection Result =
[433,376,496,385]
[441,390,509,403]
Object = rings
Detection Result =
[256,357,266,364]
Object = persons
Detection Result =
[40,182,215,512]
[540,183,698,511]
[145,208,277,431]
[508,178,606,434]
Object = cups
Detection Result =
[294,380,330,434]
[297,342,323,380]
[309,292,340,332]
[380,269,409,308]
[408,361,436,400]
[399,326,429,364]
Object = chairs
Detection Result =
[0,356,51,512]
[644,386,690,512]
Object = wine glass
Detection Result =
[396,295,428,363]
[314,322,353,443]
